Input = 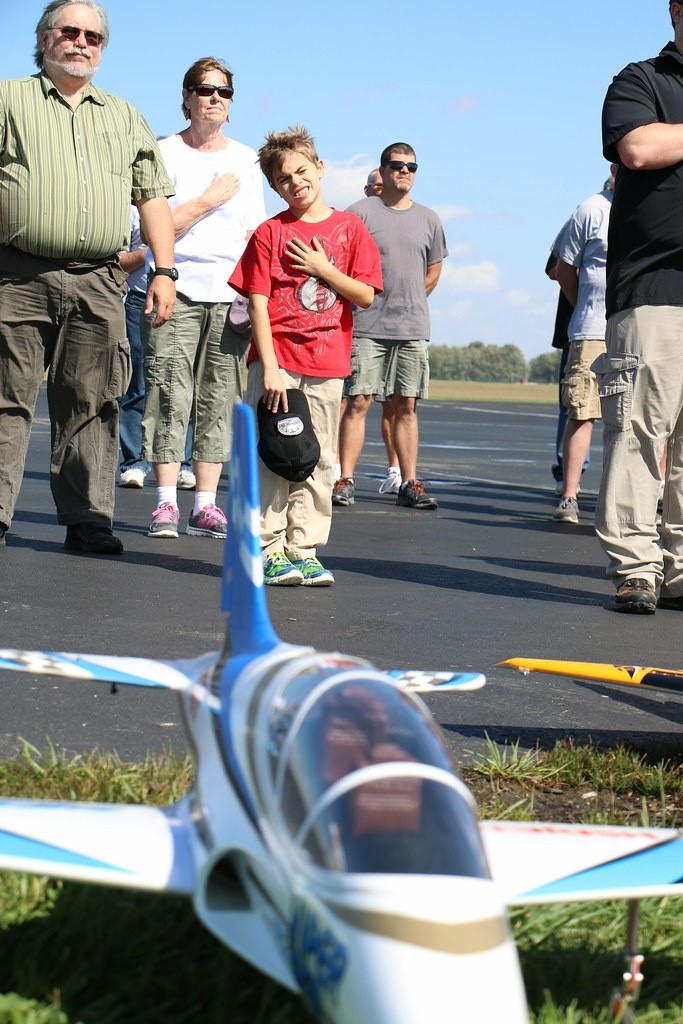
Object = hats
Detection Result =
[256,389,320,482]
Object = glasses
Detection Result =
[383,161,419,173]
[46,26,105,46]
[186,84,234,99]
[367,183,383,190]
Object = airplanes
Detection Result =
[496,655,683,696]
[0,400,682,1024]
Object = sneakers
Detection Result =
[654,512,663,525]
[657,482,665,509]
[396,479,438,509]
[555,480,585,498]
[118,468,145,488]
[613,578,656,614]
[552,496,580,524]
[657,595,683,611]
[290,557,335,586]
[263,551,304,585]
[186,503,229,538]
[379,469,403,494]
[148,501,181,538]
[332,476,357,504]
[65,521,123,554]
[0,525,6,546]
[178,469,197,490]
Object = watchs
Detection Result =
[155,267,179,281]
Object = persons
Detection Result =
[0,0,176,553]
[142,57,267,536]
[228,126,385,585]
[544,165,668,525]
[603,0,682,617]
[117,133,447,508]
